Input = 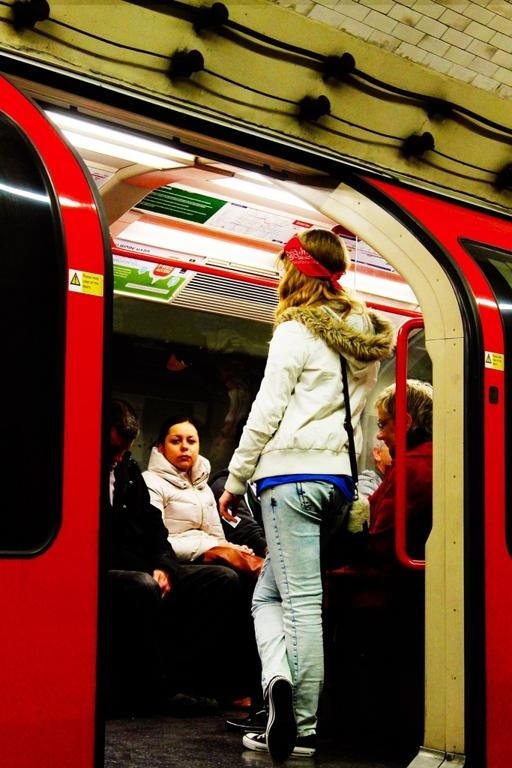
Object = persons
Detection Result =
[208,466,267,556]
[105,397,253,720]
[232,228,395,763]
[356,422,392,505]
[369,376,432,579]
[108,350,254,479]
[141,414,265,712]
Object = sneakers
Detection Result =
[226,709,274,732]
[158,693,225,719]
[265,676,299,766]
[239,724,319,759]
